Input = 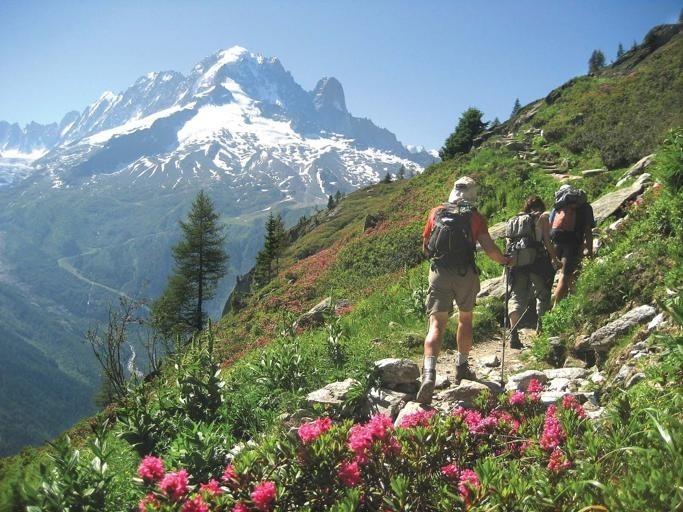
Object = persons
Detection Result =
[414,174,513,405]
[548,184,596,309]
[504,195,562,349]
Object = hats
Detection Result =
[449,177,477,204]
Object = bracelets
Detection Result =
[500,256,509,267]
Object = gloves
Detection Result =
[503,254,514,268]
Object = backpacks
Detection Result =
[505,211,544,267]
[549,206,584,242]
[424,204,476,269]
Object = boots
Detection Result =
[508,330,522,348]
[416,367,435,403]
[455,360,475,384]
[536,320,543,333]
